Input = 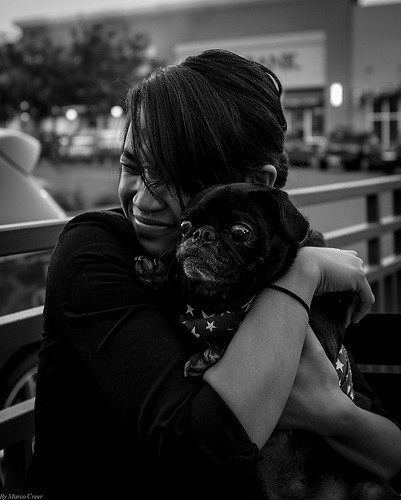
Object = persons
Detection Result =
[20,49,401,500]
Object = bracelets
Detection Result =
[265,285,311,318]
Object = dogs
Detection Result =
[170,181,355,399]
